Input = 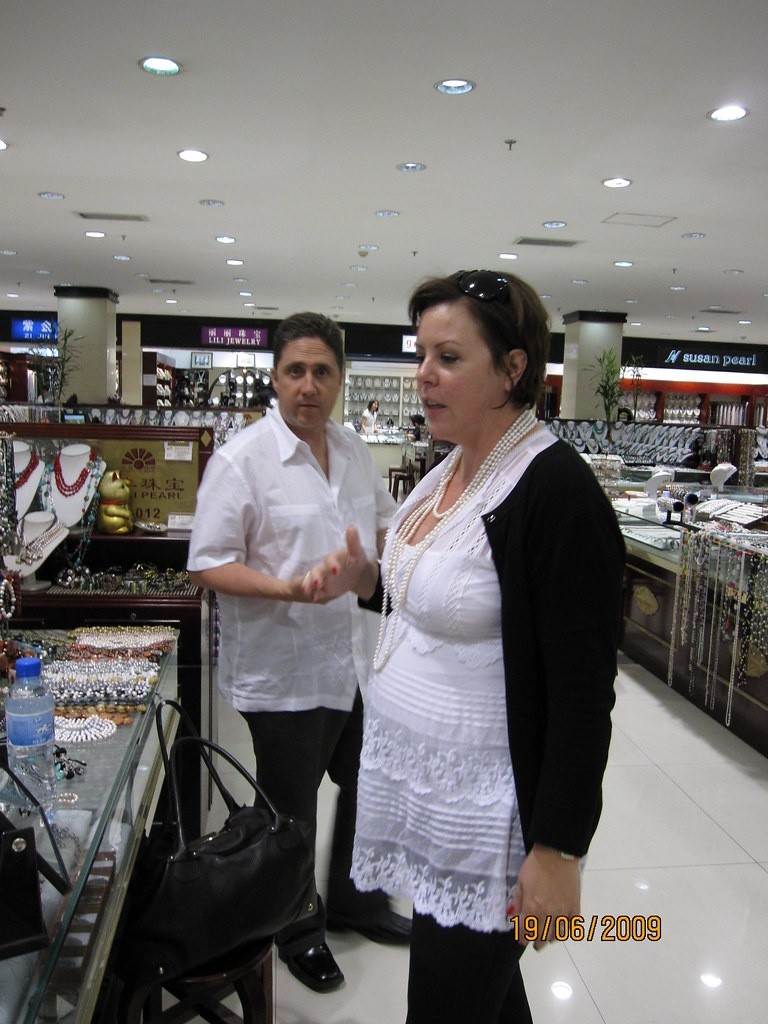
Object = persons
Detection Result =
[362,399,379,433]
[407,414,426,441]
[187,310,413,994]
[304,269,628,1024]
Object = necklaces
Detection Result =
[373,410,537,671]
[0,411,248,1024]
[345,375,453,456]
[550,390,768,728]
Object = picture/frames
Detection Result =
[191,351,214,370]
[236,353,256,368]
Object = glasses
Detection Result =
[446,269,520,346]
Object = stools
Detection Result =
[392,472,414,503]
[113,932,274,1024]
[388,467,407,495]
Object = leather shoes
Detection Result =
[276,938,345,995]
[326,907,413,946]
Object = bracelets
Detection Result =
[0,579,16,619]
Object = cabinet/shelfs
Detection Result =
[620,405,659,422]
[0,350,16,403]
[403,389,428,418]
[349,386,400,418]
[142,351,176,407]
[175,368,209,407]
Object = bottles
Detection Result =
[4,658,57,823]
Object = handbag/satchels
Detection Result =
[0,823,47,961]
[146,700,317,995]
